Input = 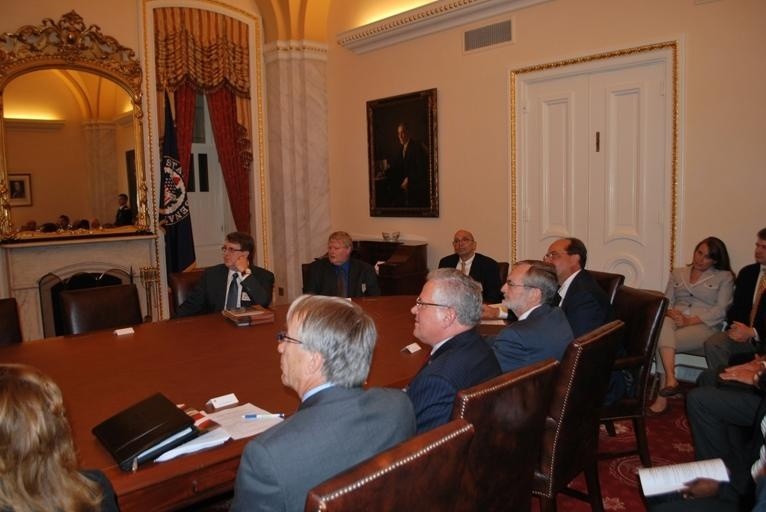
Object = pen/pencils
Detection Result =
[242,414,284,417]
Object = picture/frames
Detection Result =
[7,174,33,208]
[366,89,439,217]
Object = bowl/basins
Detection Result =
[382,232,402,243]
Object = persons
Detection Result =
[686,348,766,456]
[0,363,123,512]
[90,217,101,229]
[303,232,380,299]
[173,232,274,319]
[702,228,766,368]
[645,236,735,417]
[542,237,612,336]
[406,267,502,435]
[380,121,430,198]
[11,180,25,198]
[490,260,575,373]
[68,220,78,230]
[229,293,416,512]
[438,230,502,302]
[26,221,37,231]
[114,194,132,226]
[58,215,70,230]
[646,404,766,512]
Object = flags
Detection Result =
[157,88,196,277]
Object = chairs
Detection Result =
[451,359,561,512]
[532,319,625,512]
[59,284,143,336]
[649,321,728,400]
[168,270,208,314]
[583,269,625,305]
[598,285,670,469]
[305,419,476,512]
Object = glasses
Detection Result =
[506,280,538,289]
[416,297,449,308]
[455,238,472,243]
[543,252,571,260]
[222,247,244,253]
[277,331,303,345]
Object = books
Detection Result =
[637,455,731,496]
[91,391,284,472]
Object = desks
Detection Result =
[0,295,509,512]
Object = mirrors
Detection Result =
[0,10,151,243]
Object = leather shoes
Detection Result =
[660,383,681,397]
[648,402,672,417]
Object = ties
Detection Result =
[337,268,344,299]
[750,269,766,327]
[462,261,466,274]
[226,273,239,310]
